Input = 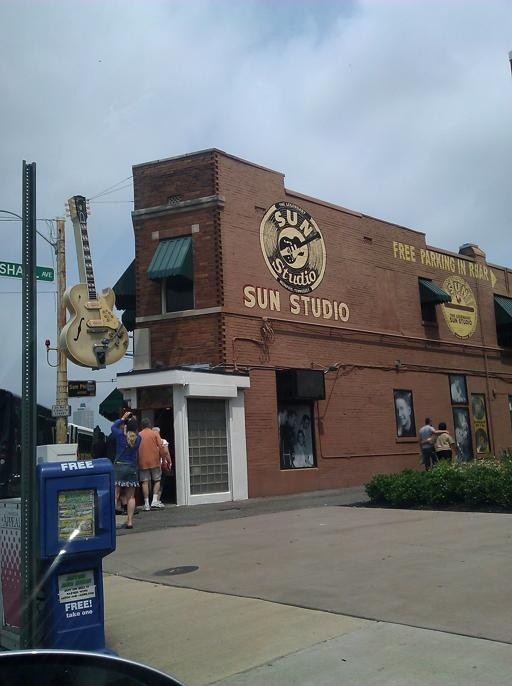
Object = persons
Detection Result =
[421,423,459,463]
[111,413,142,529]
[418,418,449,470]
[395,391,414,436]
[152,427,168,499]
[107,419,127,515]
[280,410,313,466]
[91,432,106,459]
[451,378,465,402]
[138,417,168,511]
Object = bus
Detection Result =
[0,387,56,498]
[67,421,109,462]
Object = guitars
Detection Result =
[58,195,128,370]
[280,231,321,264]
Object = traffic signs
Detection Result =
[0,259,55,283]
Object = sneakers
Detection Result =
[151,501,165,508]
[141,504,150,511]
